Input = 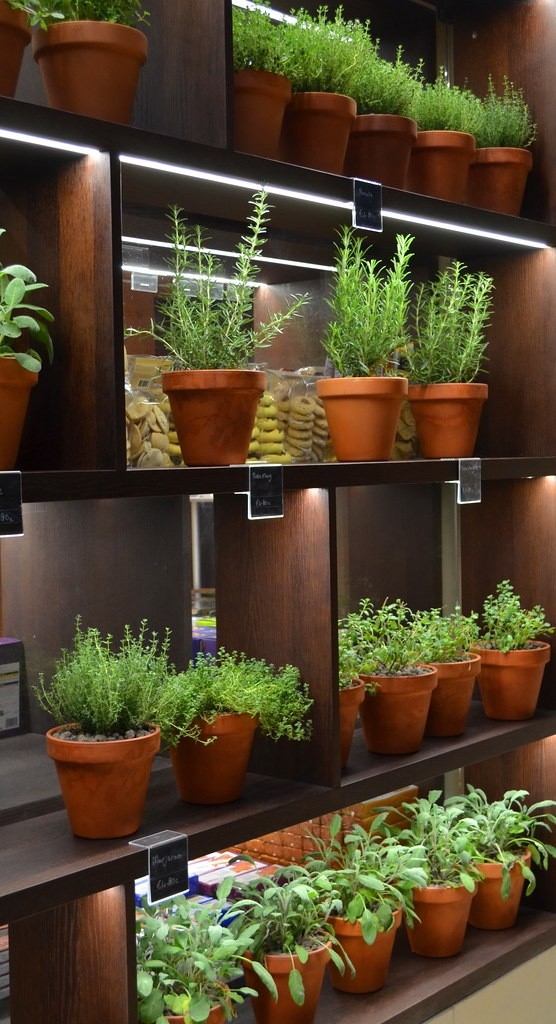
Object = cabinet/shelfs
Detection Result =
[0,0,556,1024]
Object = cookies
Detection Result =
[390,398,417,461]
[246,391,338,464]
[123,392,183,468]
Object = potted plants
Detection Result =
[33,0,151,124]
[133,792,556,1024]
[0,226,58,469]
[126,204,493,467]
[232,0,538,218]
[0,0,65,99]
[36,577,552,838]
